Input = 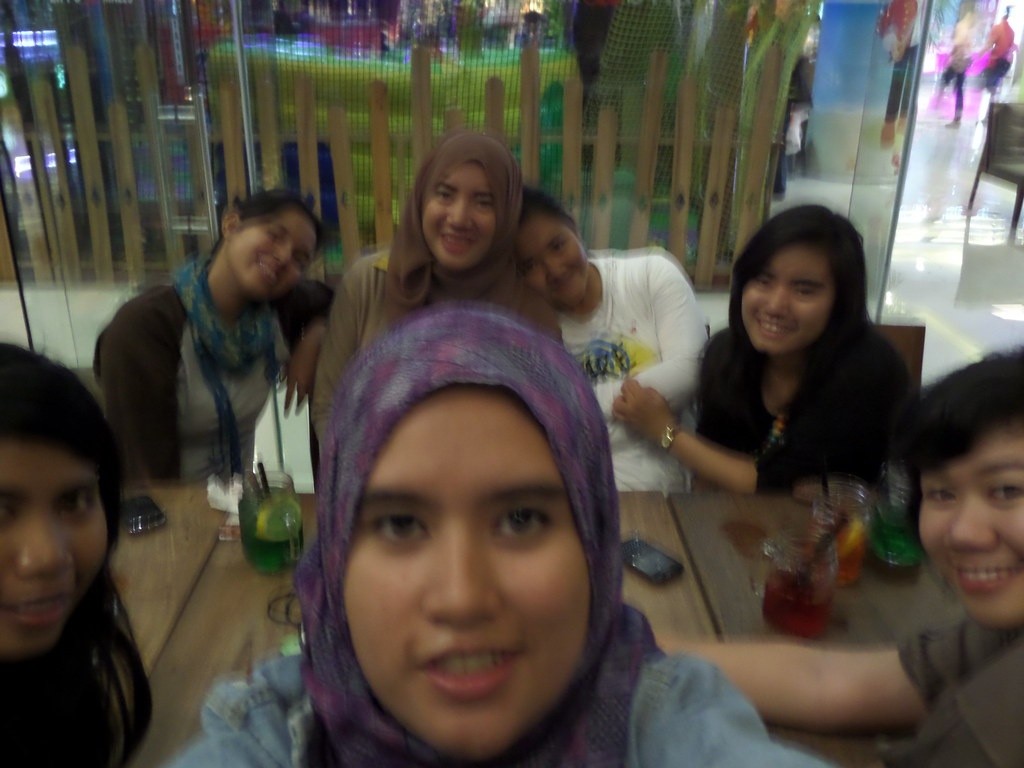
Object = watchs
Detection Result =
[659,420,685,452]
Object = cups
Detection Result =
[241,471,303,572]
[812,480,867,582]
[869,475,921,576]
[764,528,838,639]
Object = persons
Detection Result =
[612,204,917,497]
[770,55,813,205]
[310,127,564,445]
[0,342,151,768]
[164,306,830,767]
[511,183,710,492]
[93,189,334,481]
[927,0,1024,127]
[660,345,1024,767]
